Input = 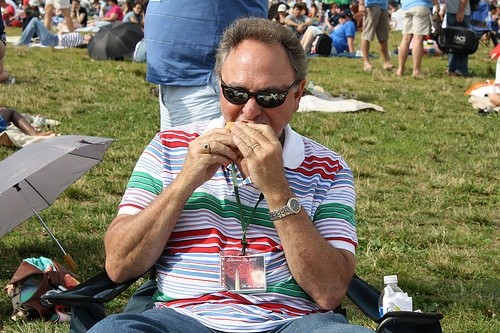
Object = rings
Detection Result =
[203,142,210,154]
[251,143,260,150]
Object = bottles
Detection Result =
[378,275,404,318]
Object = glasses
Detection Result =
[219,75,299,109]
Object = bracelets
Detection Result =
[359,4,364,5]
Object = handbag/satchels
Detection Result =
[4,256,81,321]
[435,18,478,55]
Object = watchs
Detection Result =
[268,197,303,221]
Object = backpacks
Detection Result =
[315,33,333,56]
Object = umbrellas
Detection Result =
[0,135,116,275]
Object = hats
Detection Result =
[278,4,287,12]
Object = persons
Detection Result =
[439,0,471,76]
[489,43,500,106]
[395,0,441,79]
[358,0,398,73]
[0,0,16,84]
[0,109,55,147]
[0,0,500,62]
[86,17,379,333]
[145,0,271,135]
